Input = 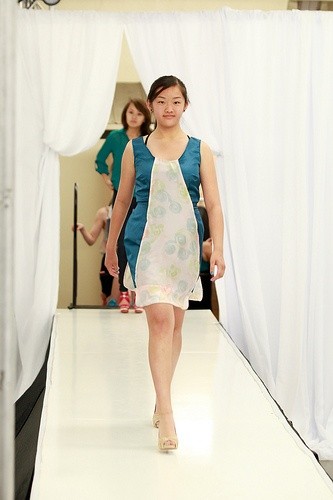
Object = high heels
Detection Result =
[157,425,178,454]
[132,292,143,314]
[118,289,130,314]
[153,405,161,428]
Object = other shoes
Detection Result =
[106,297,117,307]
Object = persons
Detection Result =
[73,196,112,307]
[94,99,155,314]
[104,75,227,453]
[188,205,212,312]
